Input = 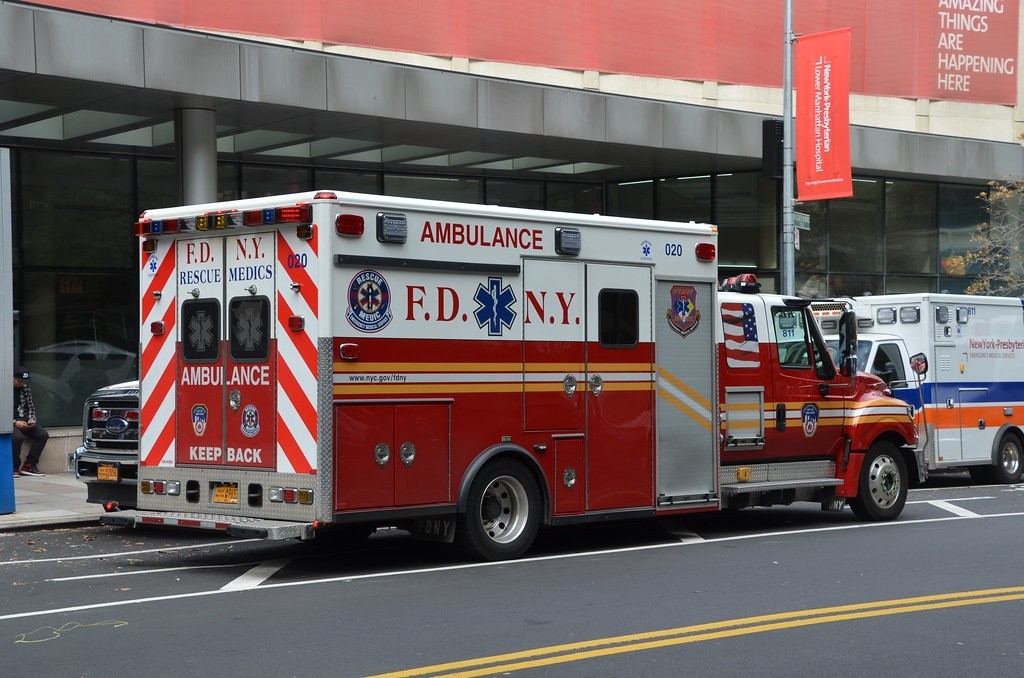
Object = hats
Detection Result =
[14,366,33,382]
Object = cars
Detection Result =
[26,342,137,380]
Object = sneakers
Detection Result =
[13,471,20,478]
[20,462,46,477]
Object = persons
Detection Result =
[13,365,49,477]
[64,352,109,427]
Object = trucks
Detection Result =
[790,292,1024,489]
[66,381,140,514]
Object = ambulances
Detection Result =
[133,191,933,563]
